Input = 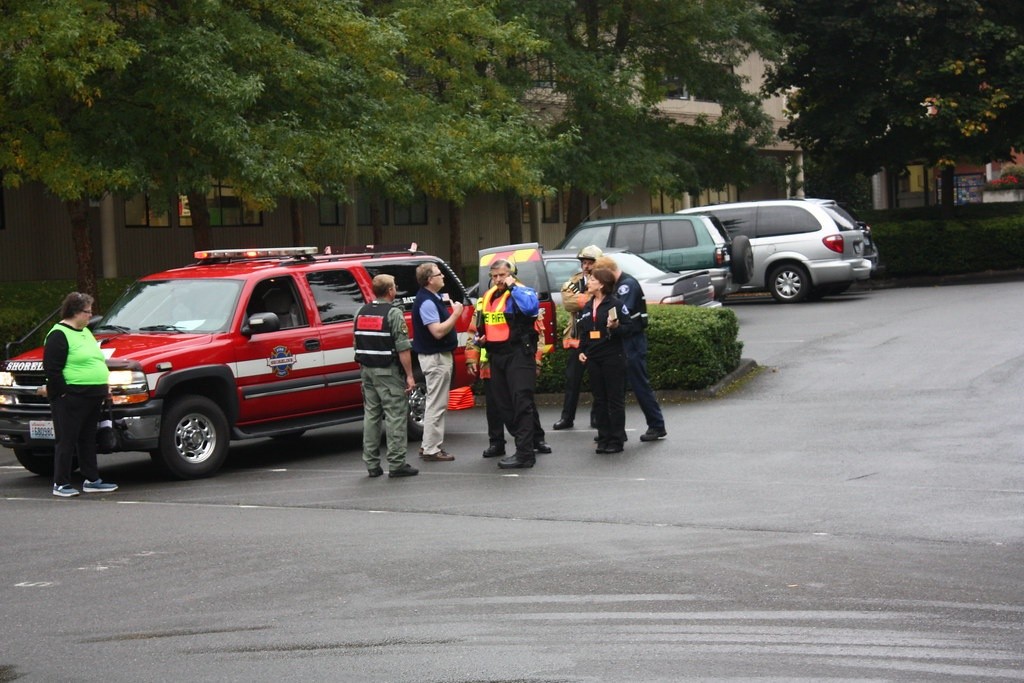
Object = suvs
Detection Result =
[638,198,880,305]
[0,242,560,483]
[546,210,756,304]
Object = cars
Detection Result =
[467,245,723,309]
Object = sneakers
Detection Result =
[640,427,667,441]
[368,466,383,477]
[53,482,80,497]
[594,432,628,441]
[83,477,119,492]
[388,463,419,477]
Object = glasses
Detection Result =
[428,272,442,278]
[80,309,93,314]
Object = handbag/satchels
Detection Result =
[95,398,118,456]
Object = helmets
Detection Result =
[576,245,604,261]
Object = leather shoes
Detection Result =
[553,418,573,430]
[498,452,536,468]
[419,447,423,456]
[483,444,505,457]
[422,450,455,462]
[533,441,552,453]
[596,443,623,454]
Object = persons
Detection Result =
[412,263,464,461]
[476,259,539,469]
[553,246,603,431]
[594,256,667,442]
[39,291,118,497]
[569,270,631,454]
[353,274,420,478]
[465,295,551,457]
